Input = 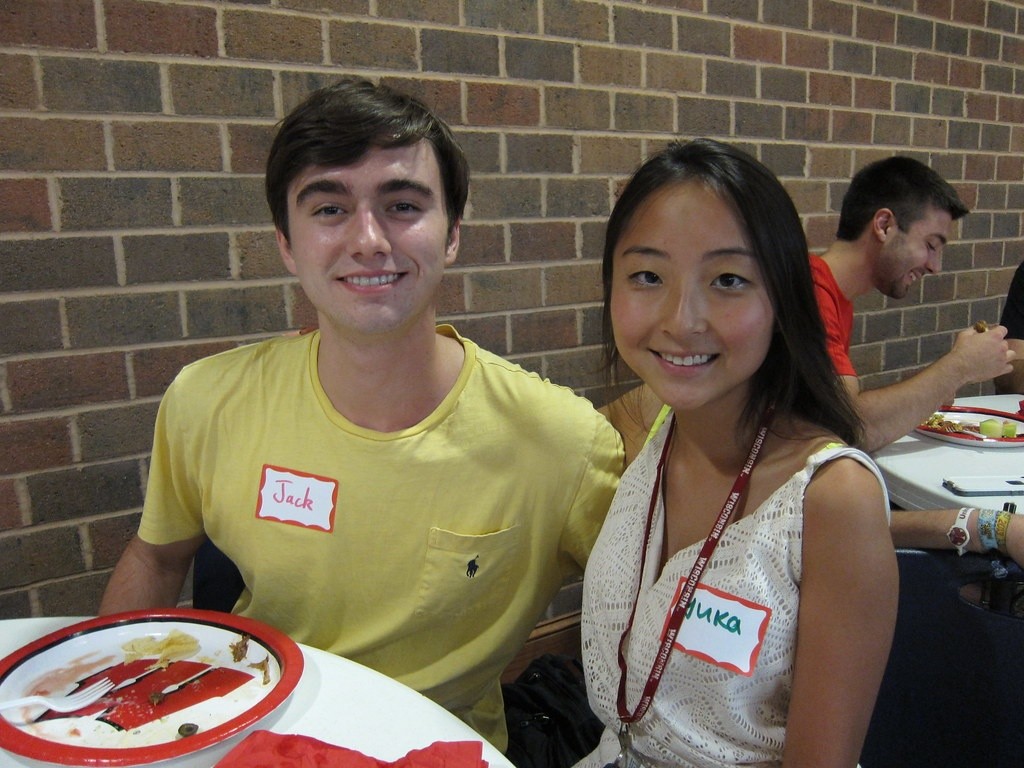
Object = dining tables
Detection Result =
[870,393,1024,512]
[0,613,515,768]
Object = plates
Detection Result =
[0,607,305,766]
[915,405,1024,448]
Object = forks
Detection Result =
[0,676,115,714]
[946,426,987,439]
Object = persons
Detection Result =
[991,260,1024,397]
[98,74,1023,759]
[803,156,1016,454]
[572,132,903,767]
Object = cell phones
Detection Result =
[942,475,1024,496]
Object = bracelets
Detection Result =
[995,510,1012,558]
[977,507,1000,552]
[947,507,976,555]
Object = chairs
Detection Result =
[861,551,1024,767]
